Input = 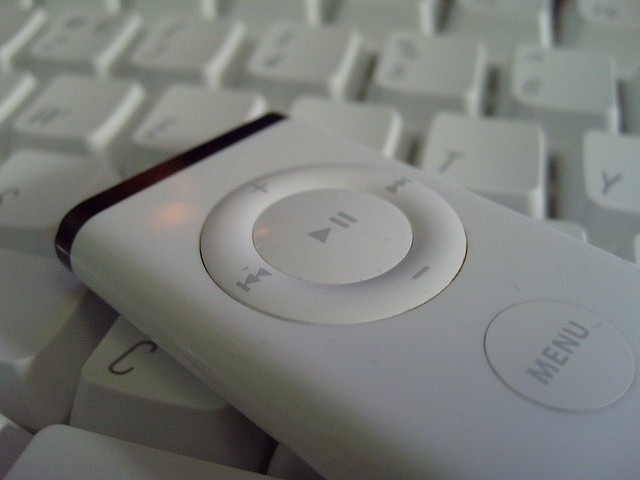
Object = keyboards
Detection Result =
[0,0,640,480]
